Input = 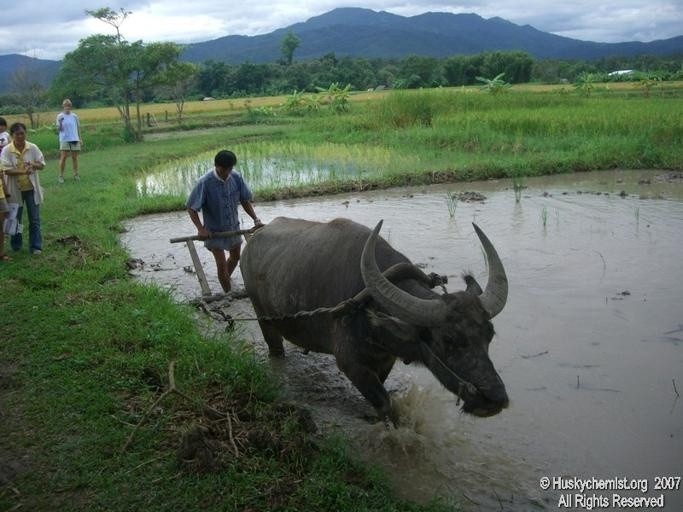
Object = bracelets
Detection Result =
[5,194,11,198]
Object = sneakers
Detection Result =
[0,249,42,261]
[58,174,80,183]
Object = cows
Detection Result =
[239,218,510,426]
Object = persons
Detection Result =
[185,150,262,294]
[0,163,15,263]
[55,98,84,184]
[0,117,12,157]
[0,122,47,255]
